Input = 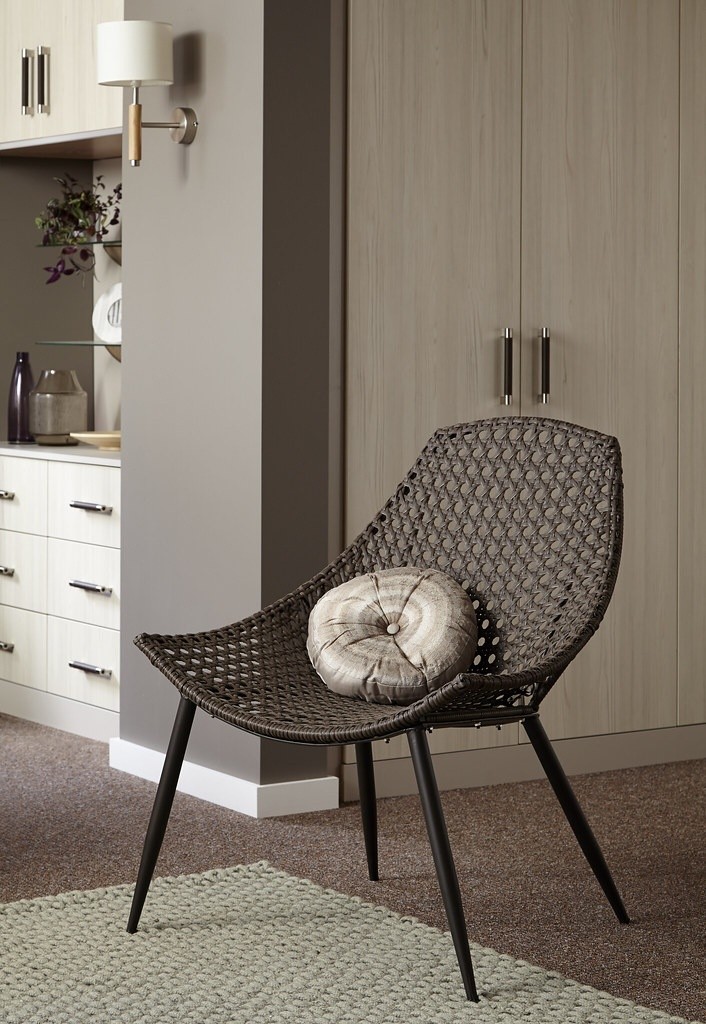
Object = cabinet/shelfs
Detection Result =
[0,0,126,162]
[0,450,120,742]
[33,240,124,363]
[340,0,706,771]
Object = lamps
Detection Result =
[90,18,198,169]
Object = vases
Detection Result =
[6,349,35,444]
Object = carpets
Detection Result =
[0,859,706,1024]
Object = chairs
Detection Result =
[105,391,635,1004]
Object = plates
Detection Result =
[71,430,121,451]
[91,281,121,344]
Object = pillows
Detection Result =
[301,550,474,704]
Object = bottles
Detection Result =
[28,369,89,445]
[8,352,33,444]
